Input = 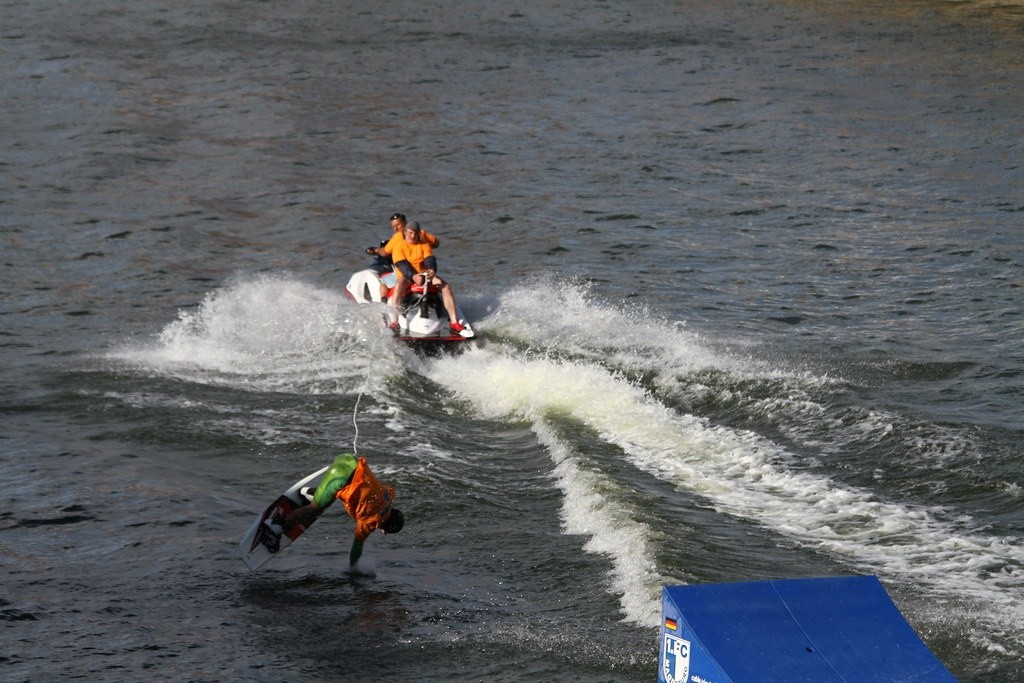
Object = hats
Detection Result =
[406,221,421,233]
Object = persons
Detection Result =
[366,214,466,333]
[283,453,404,567]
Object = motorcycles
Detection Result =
[342,239,475,343]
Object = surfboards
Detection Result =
[239,465,331,572]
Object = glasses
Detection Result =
[390,213,400,221]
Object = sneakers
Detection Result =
[300,487,317,509]
[264,517,282,536]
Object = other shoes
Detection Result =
[389,323,401,331]
[450,323,465,332]
